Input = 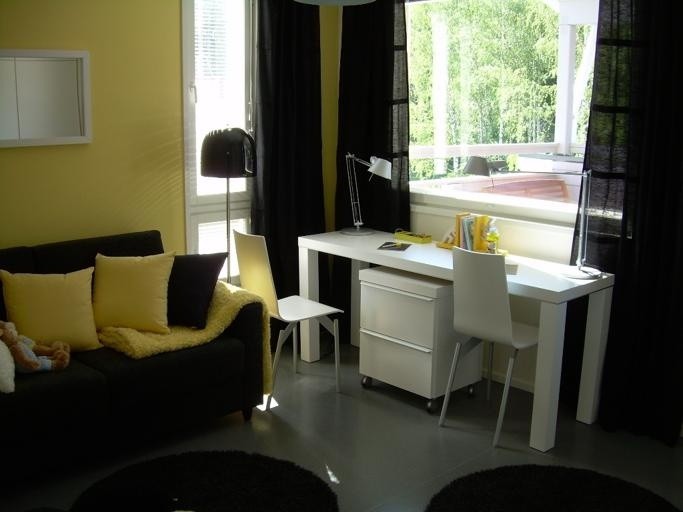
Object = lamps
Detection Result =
[458,155,602,281]
[341,148,393,237]
[200,127,256,287]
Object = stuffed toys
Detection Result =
[0,320,70,373]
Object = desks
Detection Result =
[296,225,616,452]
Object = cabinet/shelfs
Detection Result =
[351,266,485,415]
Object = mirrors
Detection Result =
[0,48,92,150]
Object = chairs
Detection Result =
[438,240,541,447]
[229,228,343,412]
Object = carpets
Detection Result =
[63,449,681,512]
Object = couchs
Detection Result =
[132,491,136,491]
[0,229,264,490]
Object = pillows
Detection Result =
[1,247,227,353]
